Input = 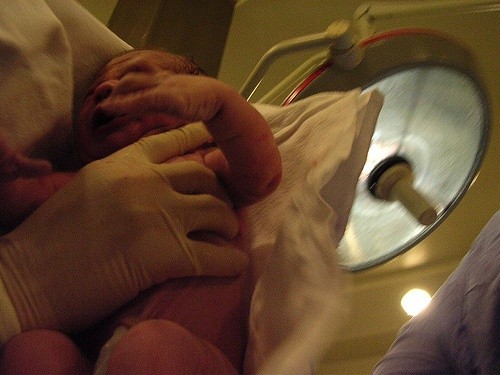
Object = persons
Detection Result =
[0,0,250,375]
[0,47,282,375]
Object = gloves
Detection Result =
[0,117,253,335]
